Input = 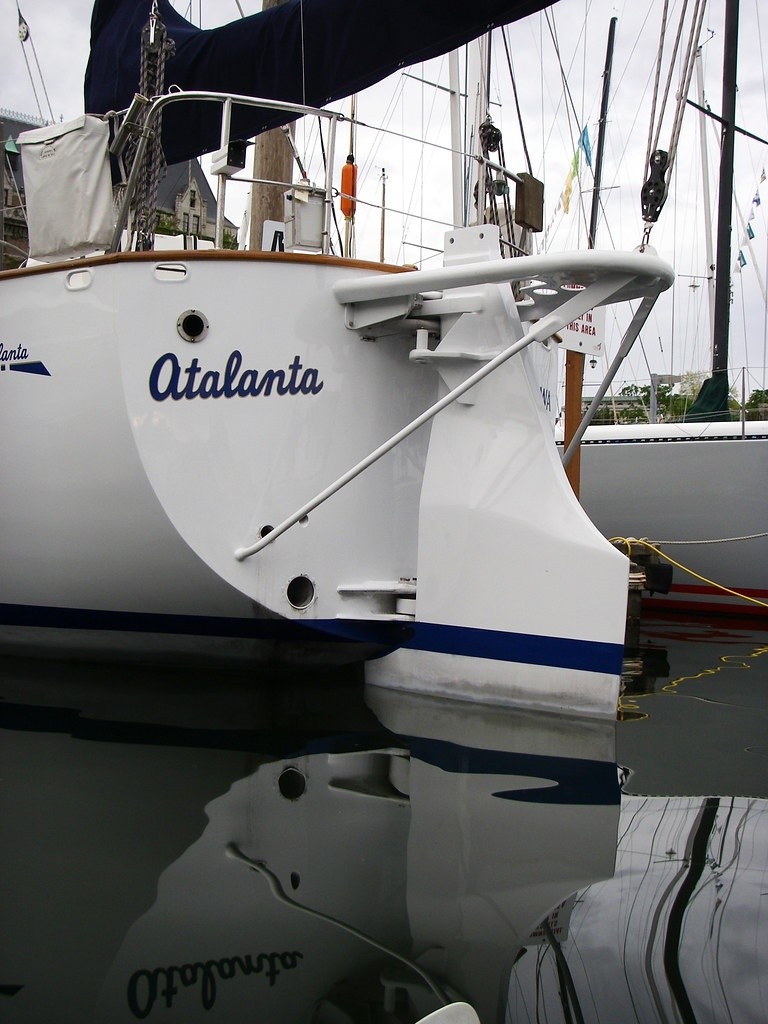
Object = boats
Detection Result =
[0,1,768,724]
[0,727,629,1024]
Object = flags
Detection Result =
[578,124,601,168]
